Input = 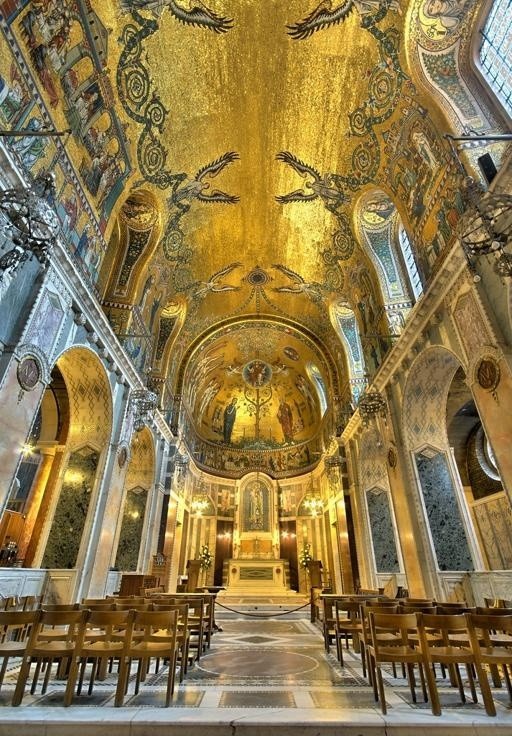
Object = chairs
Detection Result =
[324,596,512,716]
[0,596,218,706]
